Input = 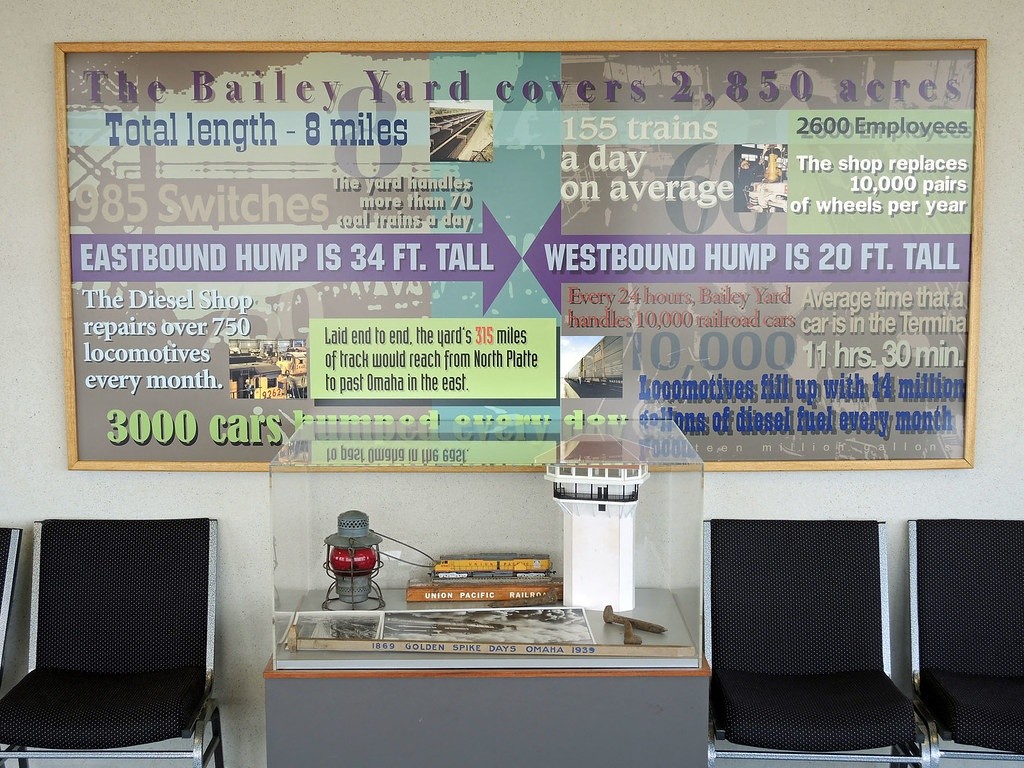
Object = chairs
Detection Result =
[0,518,223,768]
[907,519,1024,768]
[700,517,929,767]
